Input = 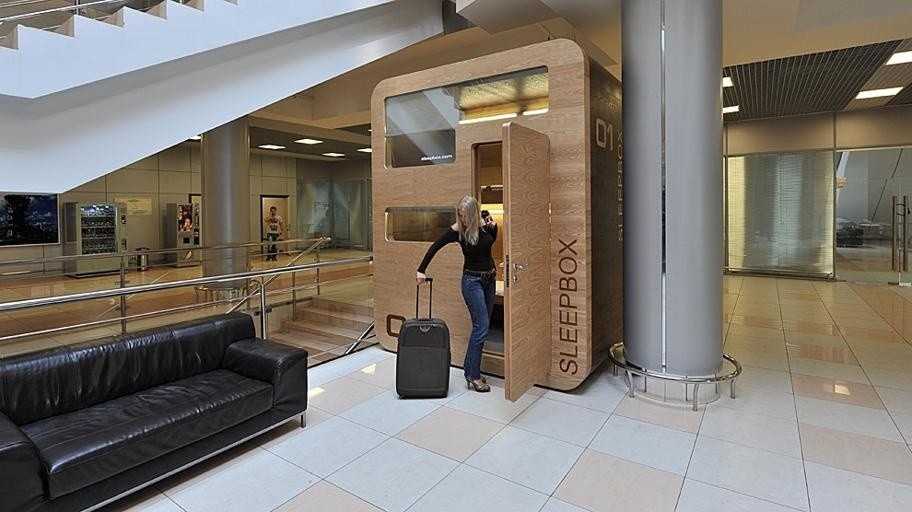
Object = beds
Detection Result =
[473,186,504,308]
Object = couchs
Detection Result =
[0,311,308,512]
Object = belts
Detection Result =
[463,269,496,279]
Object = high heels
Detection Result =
[464,373,490,393]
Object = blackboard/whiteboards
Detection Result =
[0,191,61,247]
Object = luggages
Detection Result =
[394,279,452,400]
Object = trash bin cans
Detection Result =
[136,247,150,271]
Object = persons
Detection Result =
[415,195,499,392]
[263,206,285,262]
